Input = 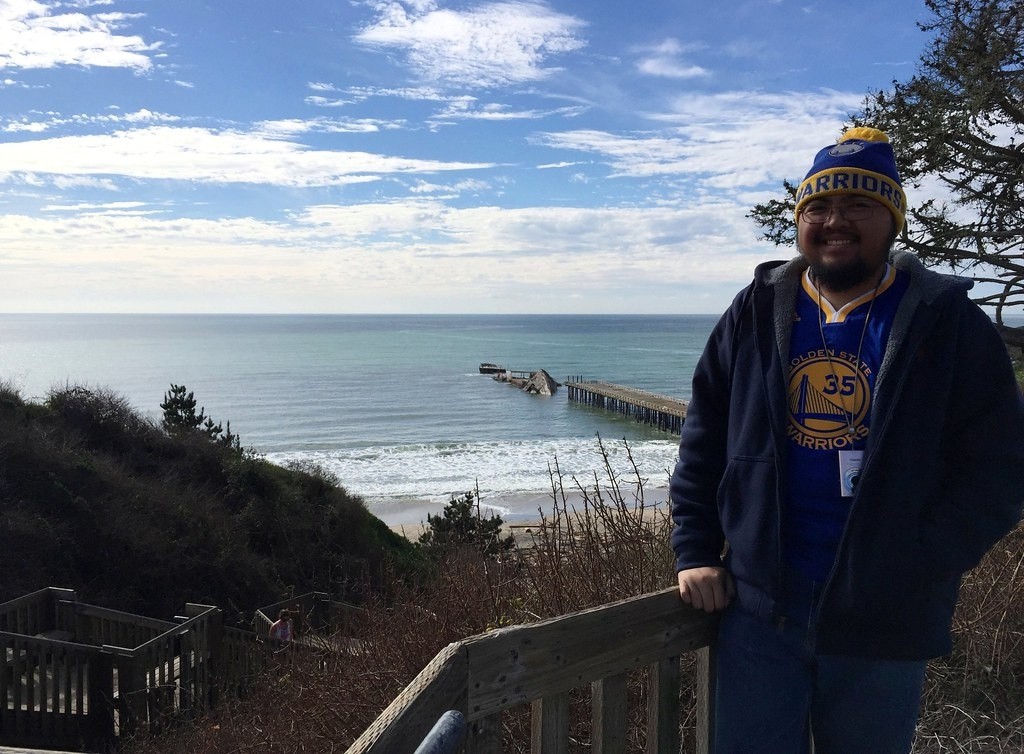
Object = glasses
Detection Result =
[798,196,883,224]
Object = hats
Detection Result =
[794,127,907,233]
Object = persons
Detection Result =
[268,609,294,686]
[669,126,1024,754]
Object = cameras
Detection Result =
[838,451,864,497]
